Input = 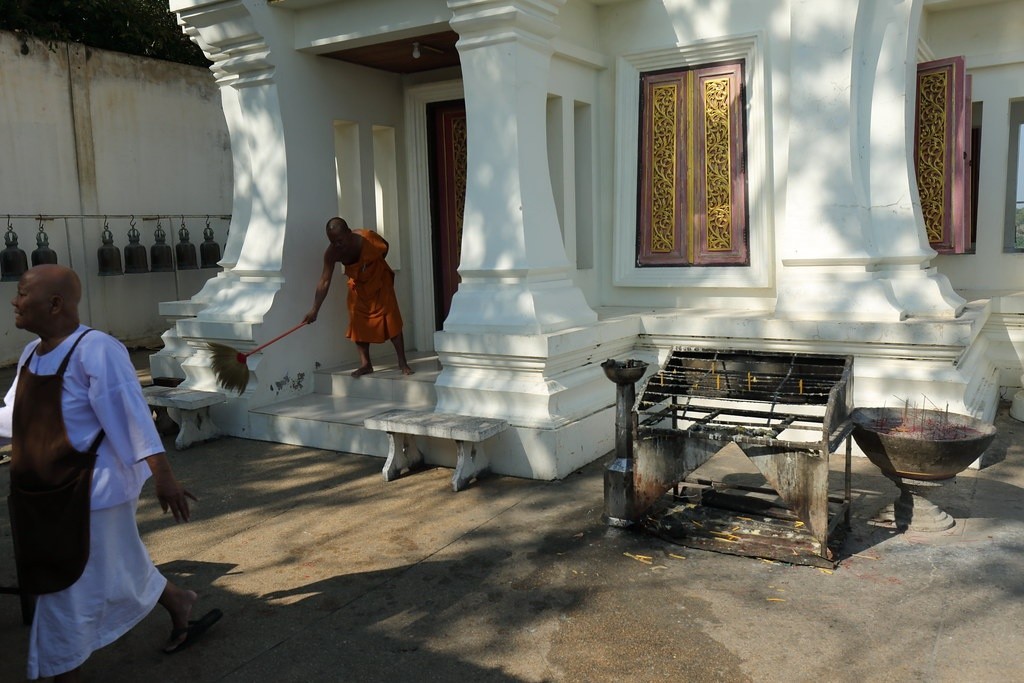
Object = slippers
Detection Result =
[163,608,223,654]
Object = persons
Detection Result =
[0,263,222,683]
[300,217,416,378]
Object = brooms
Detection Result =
[205,322,307,398]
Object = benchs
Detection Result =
[364,408,509,492]
[141,385,227,449]
